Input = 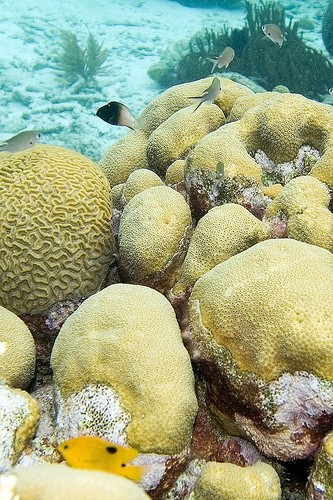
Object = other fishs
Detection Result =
[96,101,145,135]
[186,77,221,113]
[56,436,152,486]
[262,23,284,49]
[206,46,235,73]
[0,129,41,155]
